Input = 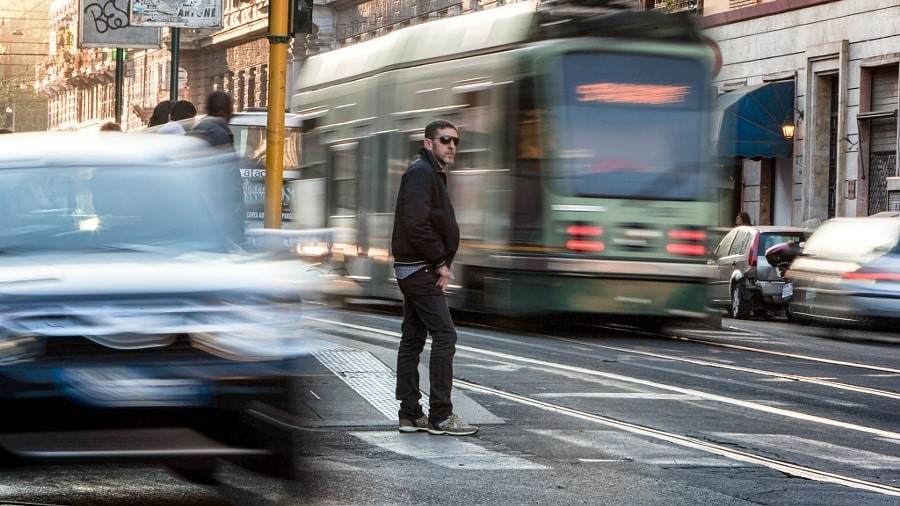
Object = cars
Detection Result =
[786,212,900,329]
[0,128,313,478]
[703,225,818,318]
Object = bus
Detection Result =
[285,1,731,343]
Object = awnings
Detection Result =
[703,76,795,160]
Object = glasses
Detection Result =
[428,136,459,145]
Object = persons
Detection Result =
[144,99,196,137]
[0,129,13,136]
[190,90,245,247]
[391,119,480,434]
[735,212,752,226]
[88,122,124,217]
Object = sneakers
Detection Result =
[399,414,429,432]
[428,413,479,435]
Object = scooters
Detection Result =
[761,238,805,323]
